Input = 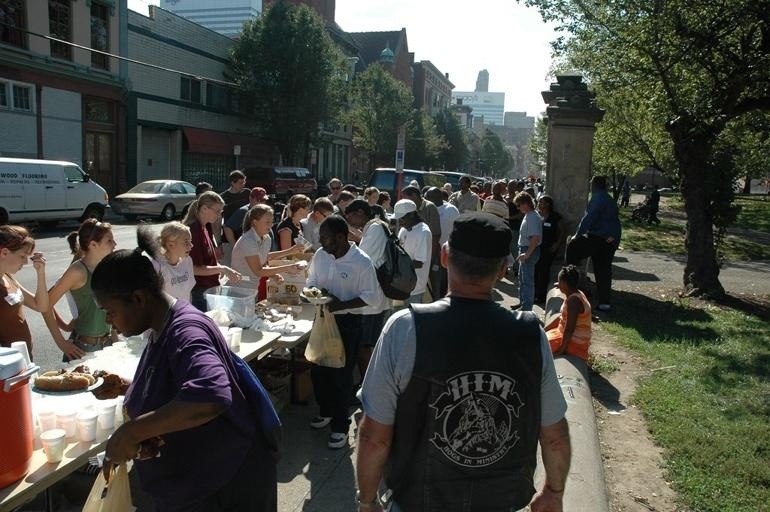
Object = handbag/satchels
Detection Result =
[229,351,286,447]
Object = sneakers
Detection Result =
[309,415,333,428]
[596,303,611,310]
[510,296,546,311]
[327,429,348,448]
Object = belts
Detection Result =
[73,330,112,346]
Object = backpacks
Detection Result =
[372,221,417,301]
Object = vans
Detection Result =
[0,156,110,229]
[245,166,319,205]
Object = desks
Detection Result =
[0,426,131,511]
[203,296,335,406]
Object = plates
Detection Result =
[307,296,331,304]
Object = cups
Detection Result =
[10,339,31,366]
[33,337,149,464]
[217,326,243,349]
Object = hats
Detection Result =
[389,198,418,219]
[449,210,512,257]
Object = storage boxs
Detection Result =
[202,285,260,329]
[265,252,312,305]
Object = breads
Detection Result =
[35,371,95,392]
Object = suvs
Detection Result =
[362,166,492,203]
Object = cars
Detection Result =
[609,183,677,193]
[113,180,200,223]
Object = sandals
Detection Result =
[76,462,102,476]
